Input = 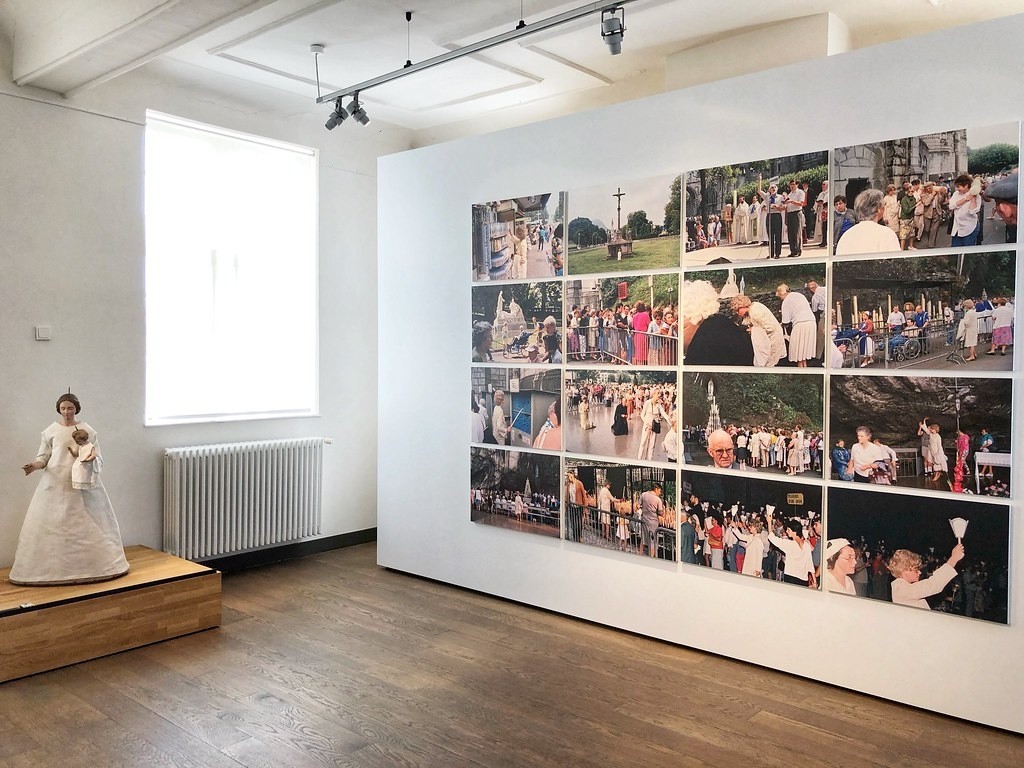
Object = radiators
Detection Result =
[156,436,335,561]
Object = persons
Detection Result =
[67,430,97,492]
[468,157,1019,621]
[22,393,104,583]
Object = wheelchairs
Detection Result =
[892,330,923,361]
[502,331,533,360]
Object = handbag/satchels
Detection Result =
[987,442,999,453]
[956,319,965,341]
[652,419,661,434]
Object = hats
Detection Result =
[826,538,850,559]
[525,344,540,354]
[923,181,932,187]
[581,395,588,399]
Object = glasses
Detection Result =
[711,448,734,456]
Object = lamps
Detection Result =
[598,7,628,55]
[325,96,350,131]
[346,89,371,127]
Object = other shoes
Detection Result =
[990,346,998,350]
[985,351,996,355]
[787,254,801,257]
[933,472,941,481]
[775,255,779,259]
[767,255,773,258]
[908,245,917,250]
[979,472,984,476]
[861,362,868,367]
[868,360,874,364]
[966,357,975,361]
[943,342,951,346]
[973,353,977,358]
[1001,352,1007,355]
[797,469,804,473]
[788,472,796,476]
[985,473,994,476]
